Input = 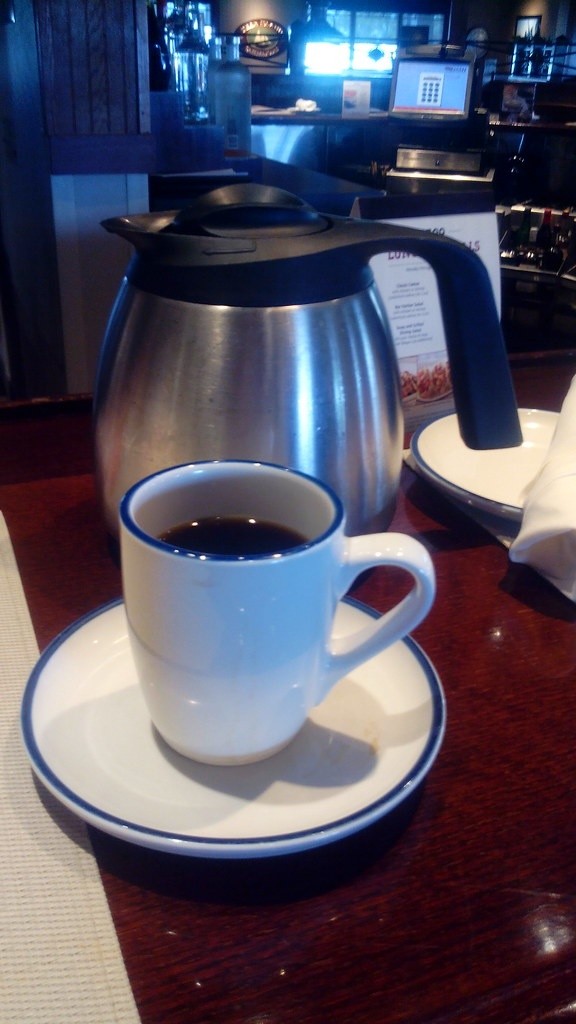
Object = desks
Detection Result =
[0,351,576,1024]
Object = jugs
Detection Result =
[93,182,525,550]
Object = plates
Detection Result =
[408,409,561,524]
[19,597,446,858]
[416,389,453,402]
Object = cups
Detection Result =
[120,458,436,766]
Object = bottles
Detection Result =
[514,208,576,295]
[148,0,253,159]
